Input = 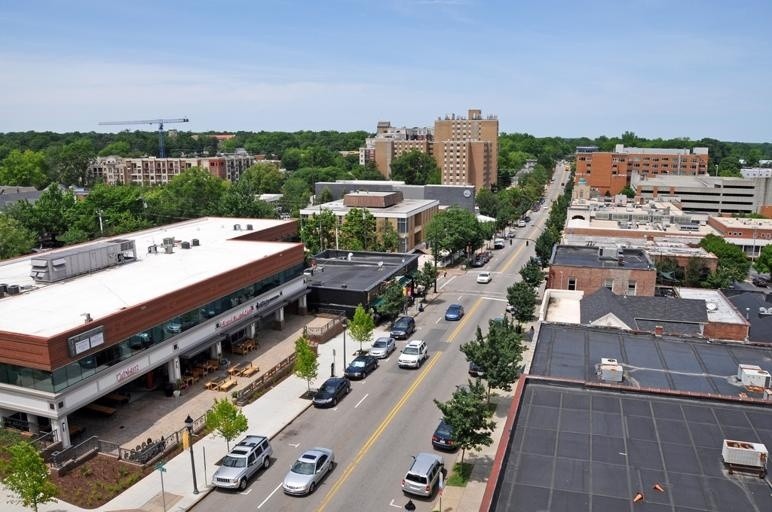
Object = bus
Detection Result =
[563,160,570,171]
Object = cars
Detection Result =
[532,197,545,213]
[505,300,514,313]
[398,383,476,501]
[496,230,517,238]
[78,291,232,371]
[281,447,335,497]
[524,216,531,222]
[470,251,494,268]
[442,303,466,324]
[753,271,770,288]
[468,359,489,378]
[490,315,510,327]
[311,277,430,409]
[476,271,492,285]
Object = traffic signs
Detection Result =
[153,461,166,471]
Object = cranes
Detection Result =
[97,117,192,159]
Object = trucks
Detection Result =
[493,238,505,249]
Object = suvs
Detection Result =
[518,221,527,227]
[210,434,275,491]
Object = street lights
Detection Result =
[154,465,166,473]
[96,207,106,234]
[402,497,442,512]
[434,234,451,295]
[183,413,199,495]
[750,221,764,267]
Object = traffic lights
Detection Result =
[526,241,529,246]
[510,240,513,245]
